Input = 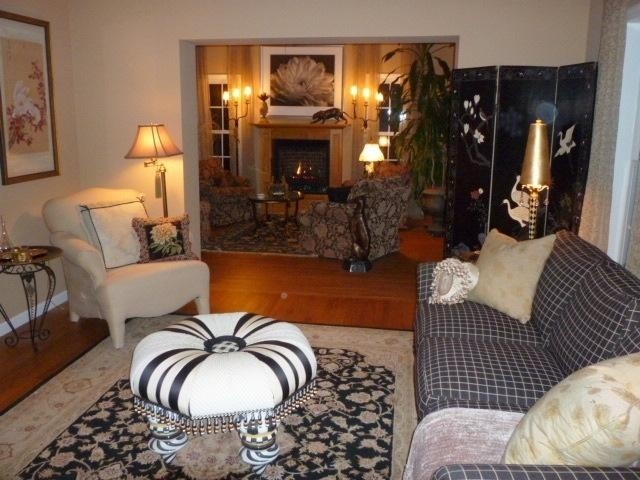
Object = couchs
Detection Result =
[403,228,640,480]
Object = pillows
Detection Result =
[498,347,640,470]
[423,228,559,327]
[75,195,201,274]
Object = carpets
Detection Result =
[198,211,320,257]
[0,312,416,478]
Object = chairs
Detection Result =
[44,189,213,350]
[296,175,412,277]
[199,158,254,227]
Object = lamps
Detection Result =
[124,120,187,221]
[516,117,555,240]
[357,143,385,178]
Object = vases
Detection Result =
[259,101,269,117]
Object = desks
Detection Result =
[0,244,65,354]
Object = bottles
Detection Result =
[0,220,15,260]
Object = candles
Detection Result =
[222,86,252,101]
[351,86,384,102]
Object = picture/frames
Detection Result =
[257,44,344,120]
[0,10,63,187]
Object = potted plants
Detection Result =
[372,40,454,240]
